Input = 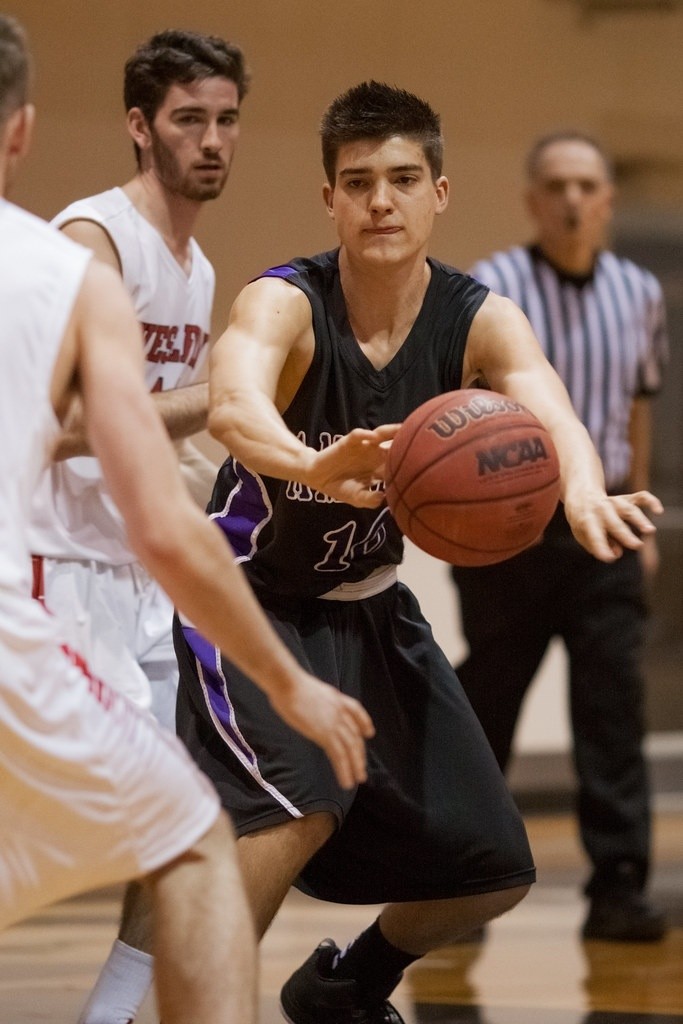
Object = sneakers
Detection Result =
[279,939,405,1024]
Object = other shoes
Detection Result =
[582,901,670,944]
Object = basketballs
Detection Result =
[385,390,559,566]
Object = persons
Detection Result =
[46,29,252,1024]
[205,79,664,1024]
[451,131,671,950]
[0,12,377,1024]
